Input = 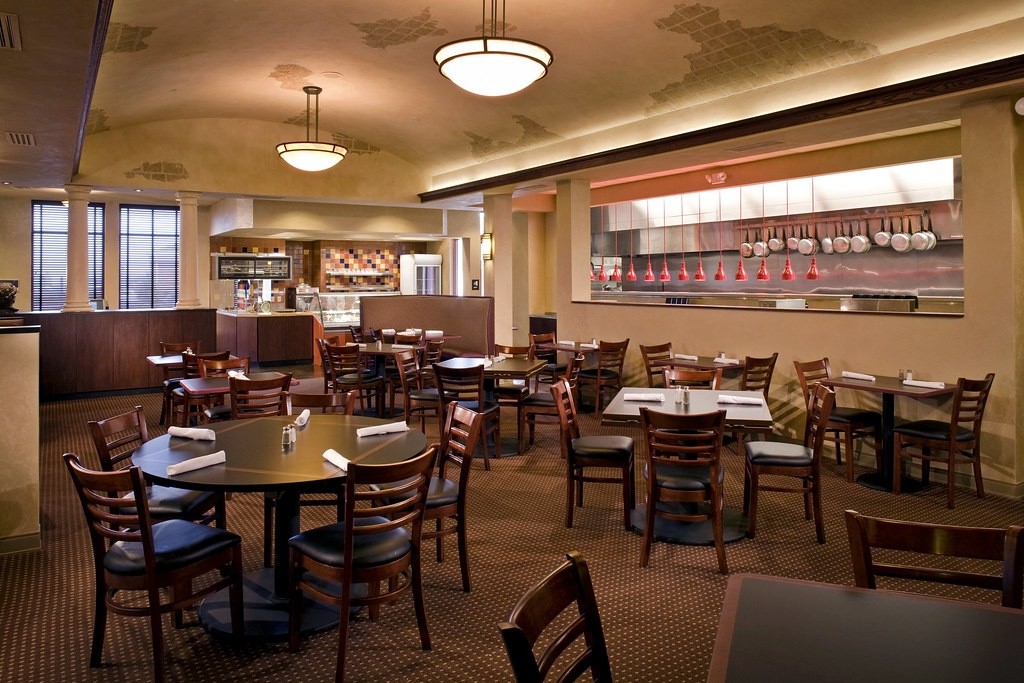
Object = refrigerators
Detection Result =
[400,254,442,295]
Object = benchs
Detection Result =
[357,294,495,358]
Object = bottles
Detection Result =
[281,427,291,444]
[682,386,690,404]
[906,369,912,380]
[717,351,725,358]
[288,424,296,442]
[186,347,192,354]
[591,338,595,344]
[490,355,495,364]
[376,338,381,348]
[484,355,488,362]
[674,385,682,404]
[898,369,904,381]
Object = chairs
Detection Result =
[739,351,1024,611]
[61,324,727,682]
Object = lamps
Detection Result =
[432,0,553,97]
[480,233,492,260]
[590,177,821,281]
[274,86,347,172]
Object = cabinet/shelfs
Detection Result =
[324,270,391,292]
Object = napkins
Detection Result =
[229,371,237,378]
[344,327,945,407]
[166,425,216,443]
[237,375,250,380]
[355,421,409,438]
[321,446,357,472]
[296,407,311,425]
[162,450,226,477]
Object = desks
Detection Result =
[337,343,426,417]
[446,355,548,454]
[600,387,774,548]
[143,351,241,417]
[819,374,954,495]
[706,574,1024,683]
[535,342,599,412]
[131,414,428,639]
[175,373,300,421]
[653,354,749,387]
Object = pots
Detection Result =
[739,213,938,258]
